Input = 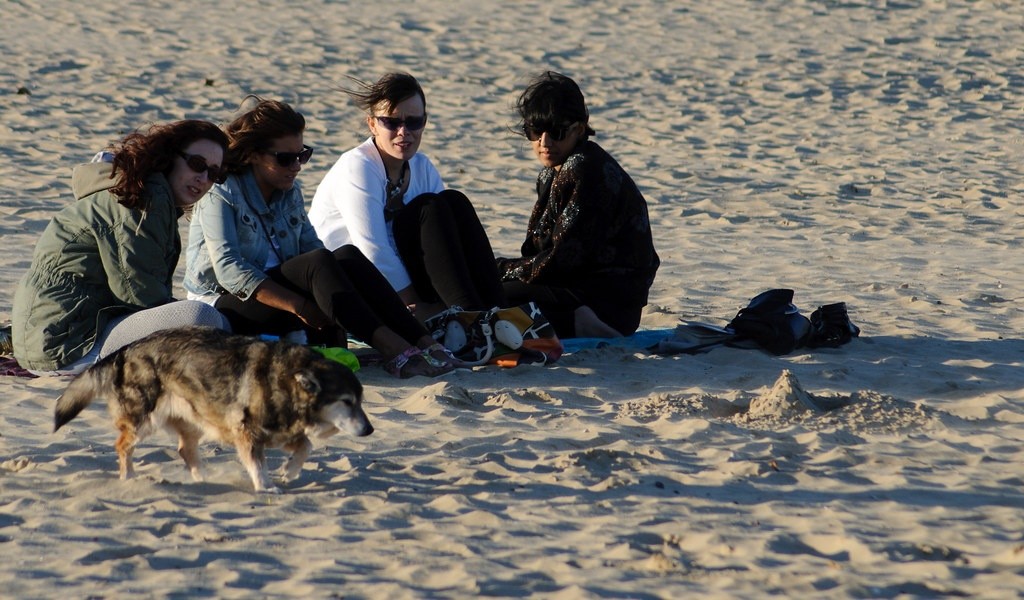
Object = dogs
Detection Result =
[52,325,374,494]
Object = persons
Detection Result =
[8,119,232,382]
[494,71,661,339]
[182,96,461,378]
[309,70,510,321]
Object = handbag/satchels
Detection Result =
[422,301,563,368]
[723,288,812,356]
[804,301,860,348]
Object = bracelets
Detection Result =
[297,299,308,319]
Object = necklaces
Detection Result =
[381,157,410,221]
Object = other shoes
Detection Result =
[421,342,465,370]
[386,346,440,379]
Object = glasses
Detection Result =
[373,112,427,131]
[522,121,575,141]
[179,152,230,184]
[264,144,313,167]
